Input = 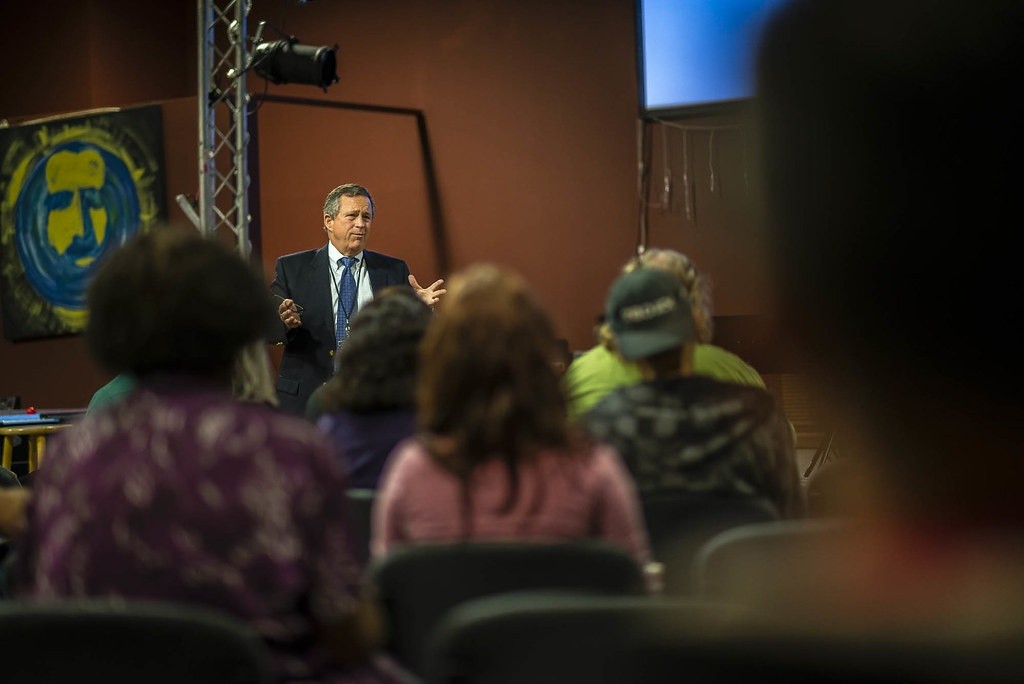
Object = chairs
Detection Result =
[0,599,278,684]
[373,536,652,680]
[689,519,849,611]
[419,590,874,684]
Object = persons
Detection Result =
[573,265,798,597]
[556,247,766,437]
[270,183,448,428]
[685,0,1024,643]
[82,285,576,571]
[368,264,652,608]
[25,227,386,684]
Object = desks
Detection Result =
[0,423,72,474]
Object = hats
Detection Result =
[608,268,694,361]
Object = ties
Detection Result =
[335,257,357,372]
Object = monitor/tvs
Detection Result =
[635,0,786,122]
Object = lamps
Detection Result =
[254,35,342,94]
